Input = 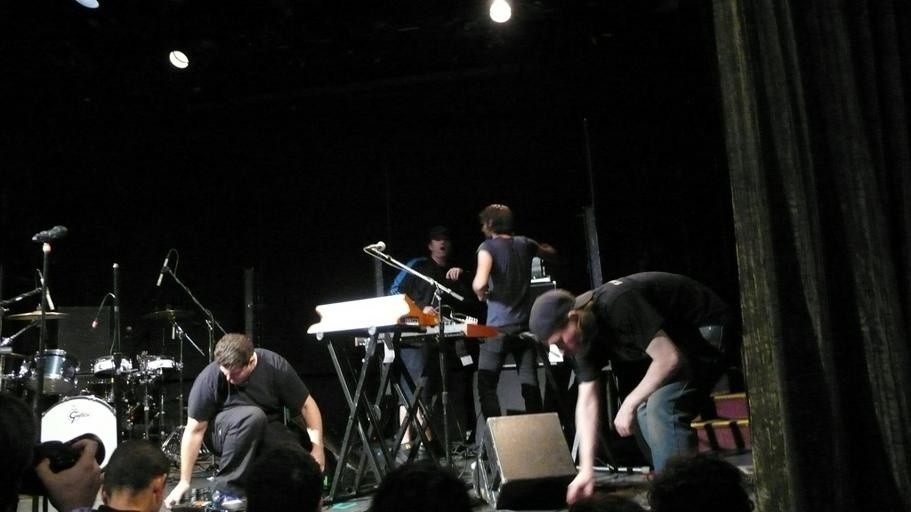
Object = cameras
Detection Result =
[18,433,105,496]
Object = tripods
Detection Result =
[136,335,210,468]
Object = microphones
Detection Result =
[36,268,56,311]
[366,241,386,251]
[156,250,172,287]
[171,317,176,340]
[32,226,68,242]
[92,315,99,329]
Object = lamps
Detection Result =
[75,0,101,10]
[168,49,190,69]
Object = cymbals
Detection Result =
[146,309,194,318]
[10,310,67,320]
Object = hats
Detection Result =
[529,289,593,339]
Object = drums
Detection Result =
[30,347,80,396]
[94,354,133,377]
[140,354,180,379]
[41,394,119,469]
[0,351,26,380]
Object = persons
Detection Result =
[388,226,466,466]
[458,204,558,460]
[242,439,326,512]
[164,331,326,510]
[95,441,172,512]
[646,451,752,511]
[368,460,486,512]
[567,493,644,512]
[526,272,725,504]
[1,439,102,512]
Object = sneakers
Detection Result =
[212,490,247,511]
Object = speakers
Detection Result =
[473,412,578,510]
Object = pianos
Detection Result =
[306,293,501,339]
